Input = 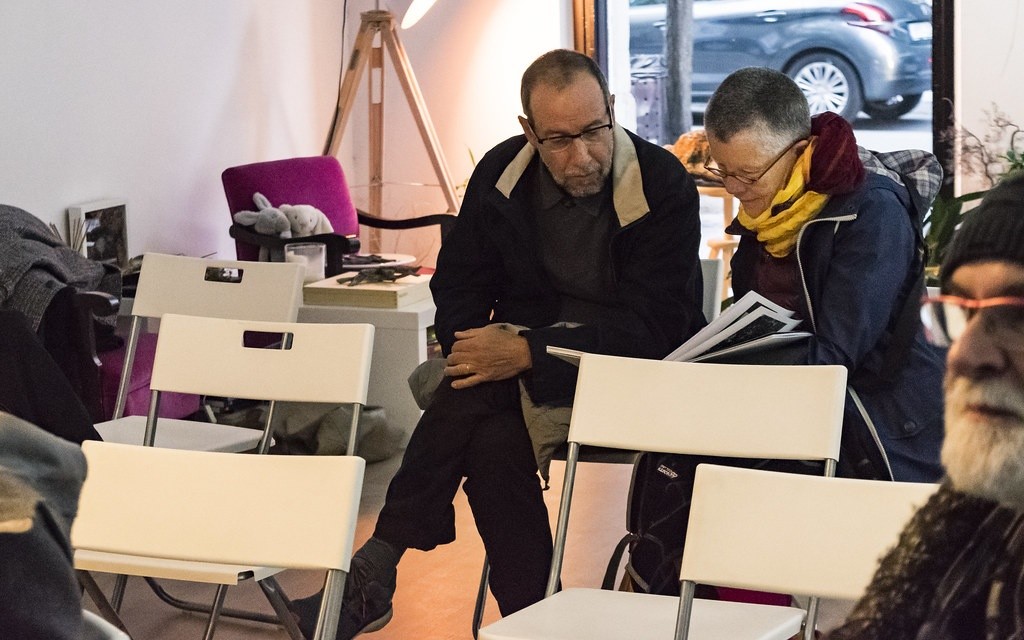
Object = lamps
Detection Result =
[322,0,461,253]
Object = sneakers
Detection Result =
[285,560,397,640]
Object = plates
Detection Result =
[342,253,417,269]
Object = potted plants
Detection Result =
[913,98,1024,350]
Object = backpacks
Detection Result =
[602,444,719,600]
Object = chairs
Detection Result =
[221,156,460,350]
[675,462,943,639]
[68,441,366,640]
[93,251,304,455]
[75,313,376,640]
[471,258,724,639]
[477,353,848,640]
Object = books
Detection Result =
[547,289,813,370]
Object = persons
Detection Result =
[620,68,945,597]
[808,162,1023,640]
[287,49,707,640]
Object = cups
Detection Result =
[285,242,326,287]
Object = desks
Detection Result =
[298,295,438,450]
[696,186,735,300]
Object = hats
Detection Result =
[933,171,1024,341]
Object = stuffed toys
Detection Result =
[279,203,334,237]
[233,192,291,263]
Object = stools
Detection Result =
[92,333,201,419]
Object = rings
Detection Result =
[466,363,470,373]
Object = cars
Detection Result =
[628,0,935,127]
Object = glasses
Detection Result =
[703,137,807,184]
[920,294,1024,355]
[527,95,613,153]
